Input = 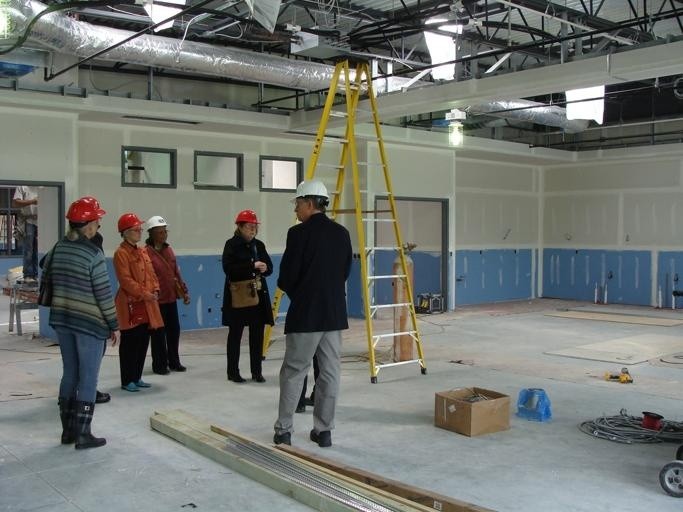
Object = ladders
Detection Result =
[261,57,428,384]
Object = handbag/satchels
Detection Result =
[228,279,259,309]
[127,300,149,326]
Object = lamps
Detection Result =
[447,120,464,149]
[142,0,186,32]
[566,85,606,126]
[424,16,463,81]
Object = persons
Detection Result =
[295,354,319,413]
[40,199,121,450]
[144,216,190,375]
[113,214,165,392]
[14,185,38,283]
[39,196,110,403]
[222,210,273,382]
[274,179,352,447]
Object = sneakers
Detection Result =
[273,431,291,446]
[310,428,331,447]
[94,364,187,403]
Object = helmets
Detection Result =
[289,180,328,203]
[234,210,260,224]
[65,197,168,233]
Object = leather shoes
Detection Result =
[251,372,266,383]
[227,373,246,382]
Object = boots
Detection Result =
[72,400,106,449]
[57,397,76,444]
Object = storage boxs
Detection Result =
[434,386,511,438]
